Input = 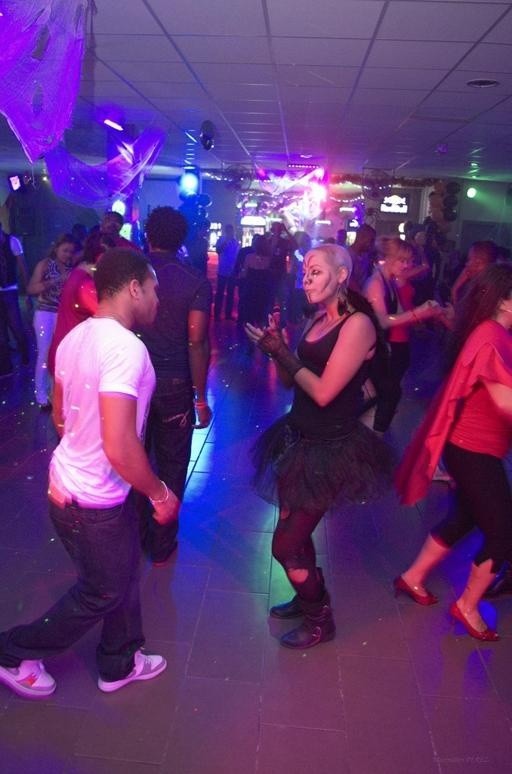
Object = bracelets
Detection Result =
[192,401,209,409]
[147,480,169,503]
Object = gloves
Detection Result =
[256,327,303,380]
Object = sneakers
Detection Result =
[153,541,178,566]
[0,658,56,699]
[97,650,167,693]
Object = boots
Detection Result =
[270,567,325,620]
[280,593,336,650]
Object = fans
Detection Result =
[362,166,394,200]
[221,162,253,195]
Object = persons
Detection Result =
[393,264,512,642]
[48,232,116,384]
[362,236,441,439]
[98,211,142,255]
[264,222,299,292]
[346,223,377,296]
[0,250,180,699]
[0,221,32,379]
[235,236,281,355]
[244,243,399,649]
[133,206,212,567]
[27,236,76,407]
[440,239,499,369]
[214,224,242,322]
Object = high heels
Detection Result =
[394,573,439,605]
[450,602,500,642]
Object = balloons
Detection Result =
[430,180,460,223]
[177,190,212,229]
